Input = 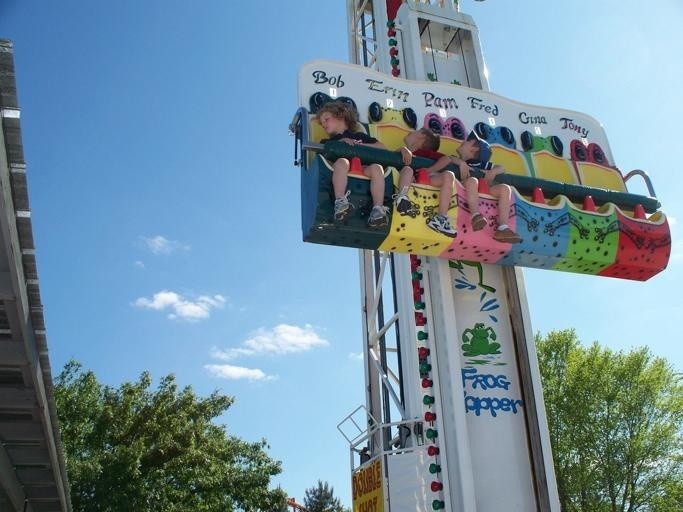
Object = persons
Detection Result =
[391,128,458,238]
[446,130,521,244]
[312,100,391,228]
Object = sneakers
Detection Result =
[428,214,457,238]
[396,194,411,212]
[367,207,389,227]
[493,228,521,243]
[334,198,355,220]
[472,214,487,230]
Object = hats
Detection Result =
[466,130,492,161]
